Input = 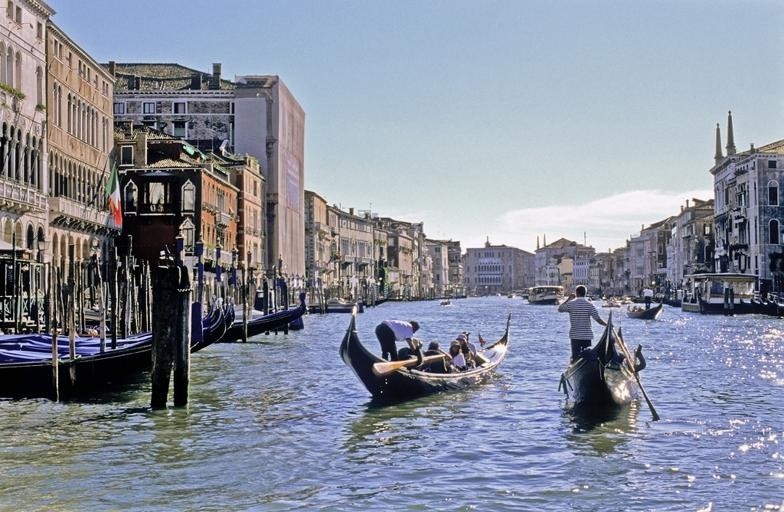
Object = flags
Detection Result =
[105,165,121,225]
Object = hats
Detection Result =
[450,341,461,349]
[456,334,468,342]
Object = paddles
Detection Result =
[372,354,447,376]
[612,327,660,422]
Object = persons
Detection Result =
[374,319,477,373]
[557,284,614,364]
[642,286,654,311]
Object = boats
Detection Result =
[330,304,516,404]
[440,301,452,306]
[496,281,666,319]
[553,307,648,414]
